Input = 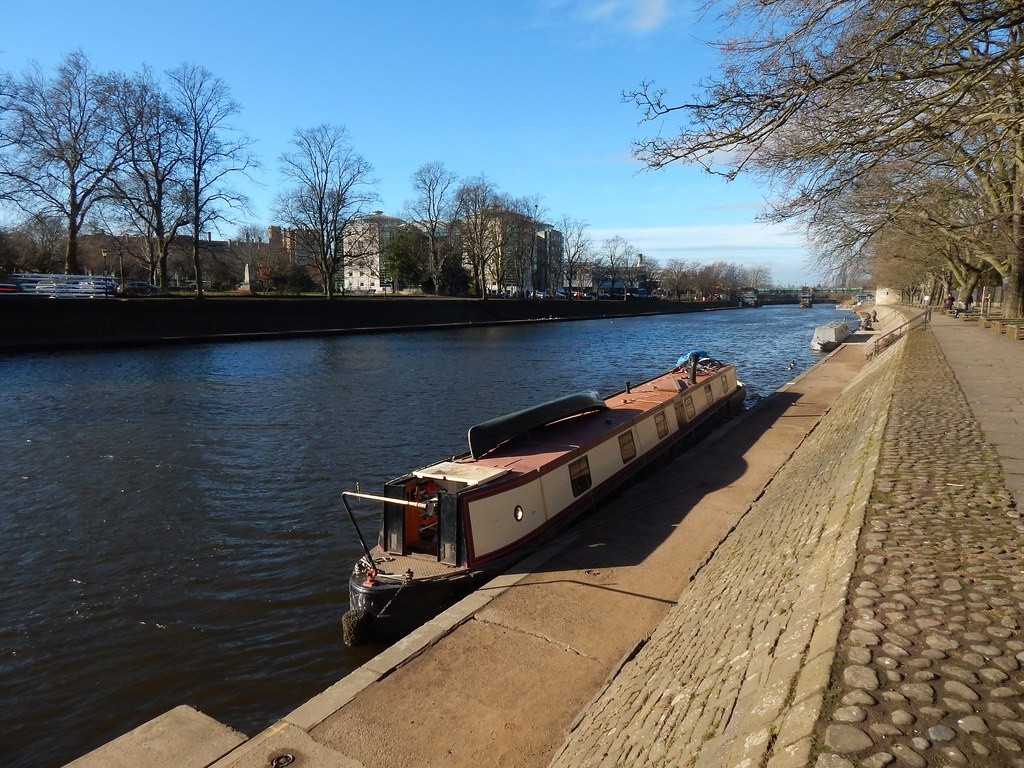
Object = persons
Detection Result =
[967,296,973,312]
[955,300,966,318]
[864,313,870,322]
[500,289,530,299]
[871,310,877,322]
[944,293,955,314]
[340,284,345,300]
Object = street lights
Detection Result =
[101,248,108,299]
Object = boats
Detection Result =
[799,289,814,309]
[349,352,747,641]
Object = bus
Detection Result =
[185,281,212,291]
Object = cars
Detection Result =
[115,280,169,296]
[529,289,597,299]
[36,281,56,294]
[0,280,20,292]
